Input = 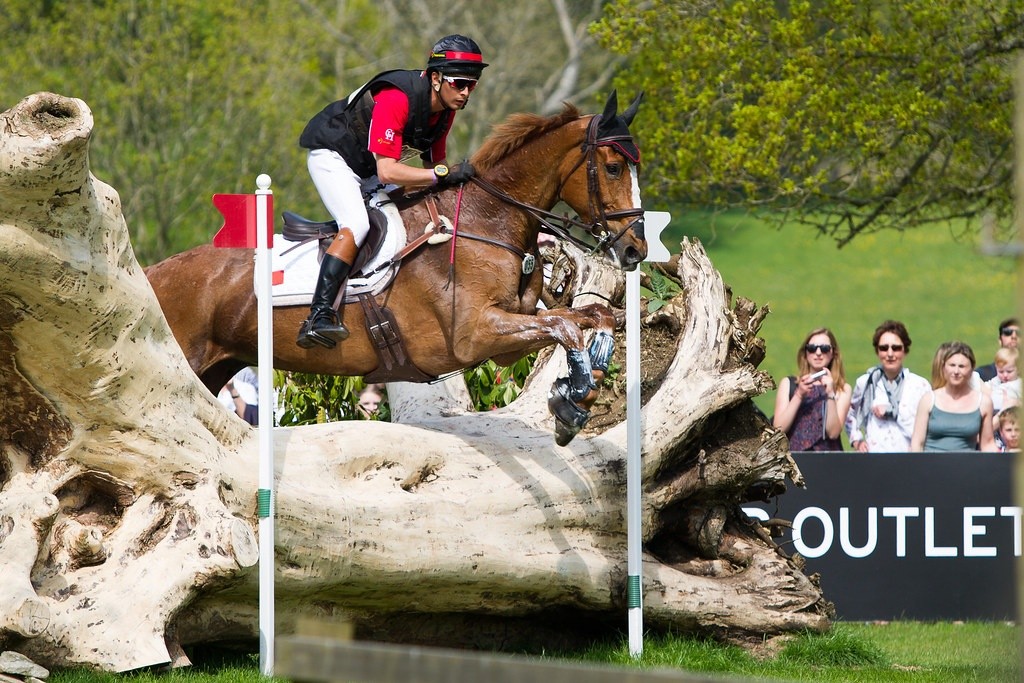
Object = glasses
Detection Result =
[1003,329,1022,337]
[878,344,902,352]
[442,74,479,90]
[805,344,832,353]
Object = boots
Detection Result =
[298,252,350,348]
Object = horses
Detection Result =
[137,89,650,448]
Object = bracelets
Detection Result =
[827,394,835,400]
[432,169,437,187]
[795,390,802,399]
[233,393,238,398]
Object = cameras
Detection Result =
[811,370,827,382]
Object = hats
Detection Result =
[428,34,490,77]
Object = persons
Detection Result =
[357,384,384,420]
[218,368,259,427]
[772,328,853,451]
[844,320,932,452]
[913,341,1024,453]
[974,318,1023,381]
[295,34,489,349]
